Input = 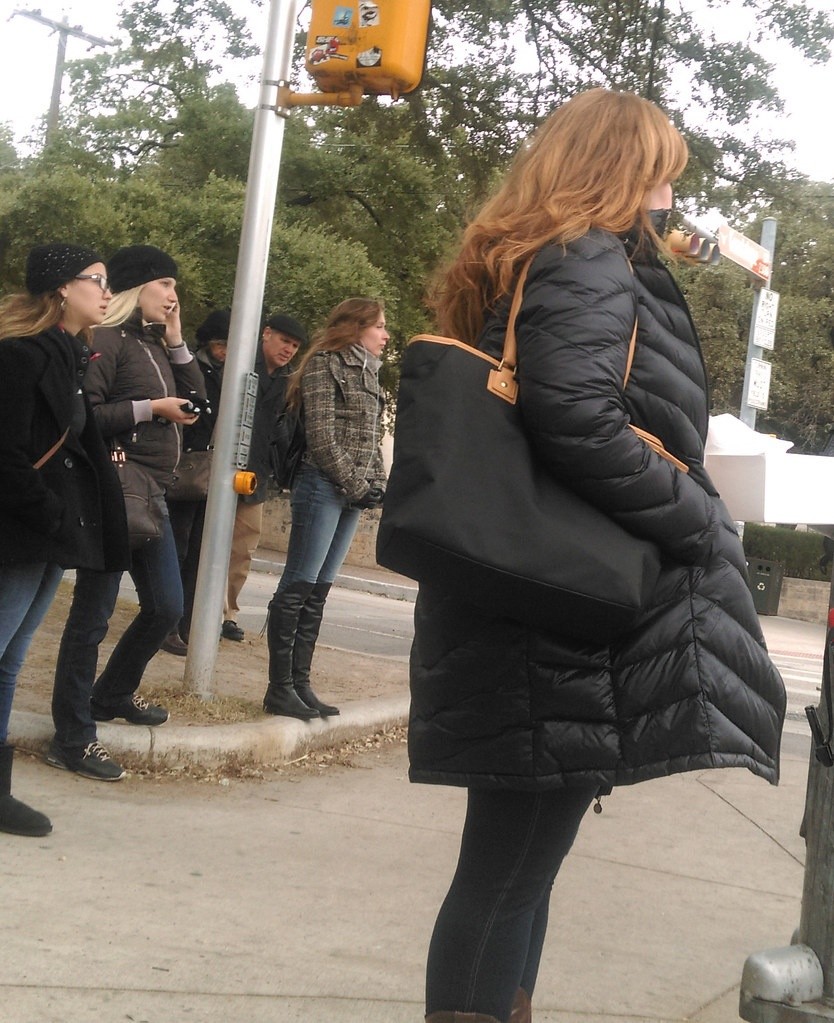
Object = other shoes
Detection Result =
[220,620,245,641]
[161,626,192,656]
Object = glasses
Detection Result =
[74,273,113,294]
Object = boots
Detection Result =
[259,580,340,718]
[0,746,53,836]
[426,987,532,1023]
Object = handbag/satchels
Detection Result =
[108,458,169,539]
[165,446,214,501]
[373,241,689,632]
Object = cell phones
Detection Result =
[169,301,176,313]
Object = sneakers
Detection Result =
[88,691,171,726]
[44,738,126,781]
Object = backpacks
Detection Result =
[269,384,306,496]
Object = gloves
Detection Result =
[351,486,384,510]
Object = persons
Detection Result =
[1,238,302,835]
[260,297,390,719]
[407,86,785,1023]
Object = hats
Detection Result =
[268,315,307,343]
[25,244,106,292]
[195,308,230,339]
[107,244,177,293]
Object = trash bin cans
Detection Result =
[746,558,784,617]
